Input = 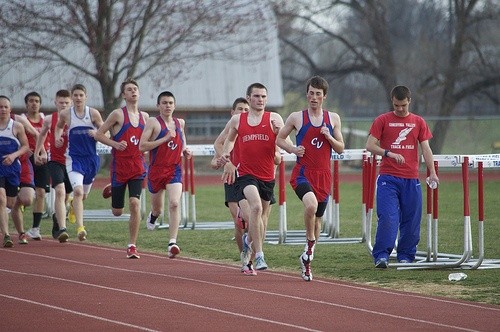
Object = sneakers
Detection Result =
[67,200,77,223]
[241,265,254,271]
[58,230,69,242]
[303,240,317,262]
[168,243,180,259]
[102,184,112,199]
[41,211,48,218]
[255,258,267,270]
[244,264,257,275]
[236,208,247,229]
[27,229,40,240]
[3,235,13,247]
[240,233,253,266]
[77,226,87,241]
[146,211,158,230]
[299,254,313,281]
[126,244,141,259]
[18,233,29,244]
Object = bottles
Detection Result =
[448,272,468,281]
[426,176,437,189]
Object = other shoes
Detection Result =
[374,258,389,268]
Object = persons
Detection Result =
[19,92,50,241]
[0,95,47,248]
[275,75,345,281]
[210,97,281,273]
[138,91,192,258]
[213,83,293,276]
[54,84,106,241]
[34,90,72,242]
[93,77,151,258]
[366,85,439,269]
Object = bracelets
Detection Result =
[218,156,229,168]
[385,149,390,158]
